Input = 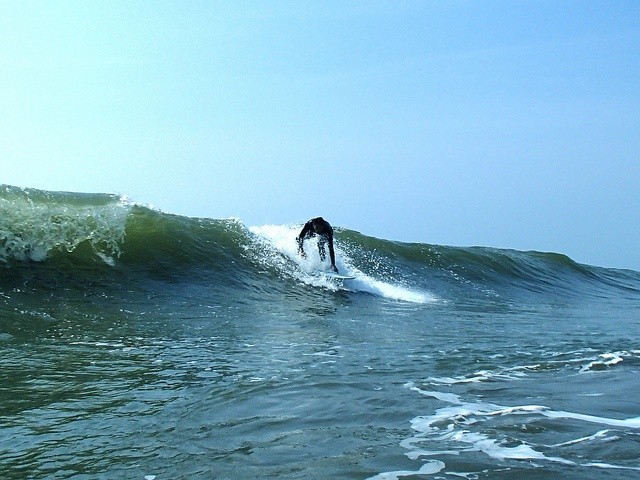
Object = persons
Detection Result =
[295,217,338,274]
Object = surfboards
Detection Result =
[309,264,354,280]
